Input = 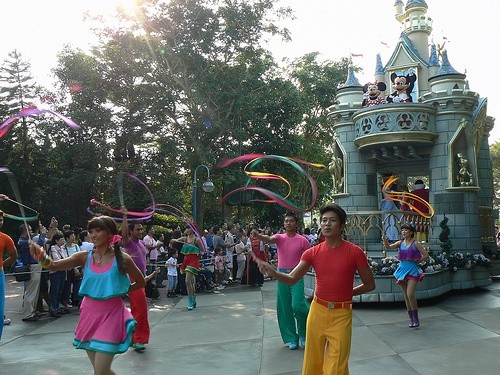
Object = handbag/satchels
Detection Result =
[71,265,82,279]
[15,265,31,282]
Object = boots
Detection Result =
[407,310,413,327]
[411,309,419,327]
[186,295,196,308]
[187,295,193,310]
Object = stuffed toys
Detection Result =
[359,67,416,130]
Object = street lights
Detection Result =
[192,164,214,224]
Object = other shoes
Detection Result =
[22,313,42,321]
[50,312,61,317]
[4,319,11,325]
[167,293,178,298]
[134,342,145,350]
[289,342,299,350]
[300,336,305,347]
[257,283,263,287]
[61,310,71,314]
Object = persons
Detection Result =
[17,216,326,321]
[120,213,161,351]
[27,215,147,375]
[0,211,17,343]
[252,212,310,351]
[170,224,206,310]
[256,204,376,375]
[381,225,430,327]
[378,176,429,241]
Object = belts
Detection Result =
[313,295,352,308]
[277,268,293,273]
